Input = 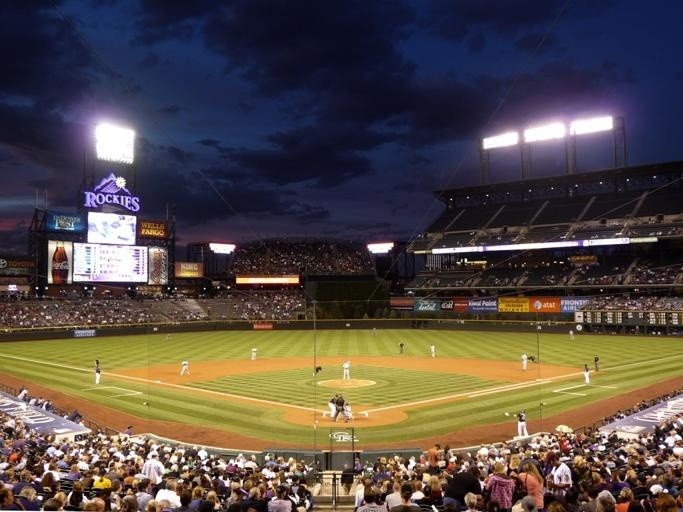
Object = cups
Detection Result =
[547,480,552,491]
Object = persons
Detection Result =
[584,355,600,384]
[251,347,257,360]
[0,385,683,512]
[94,359,102,384]
[521,352,536,370]
[0,294,303,328]
[342,360,350,380]
[180,359,191,376]
[569,328,575,341]
[417,216,683,311]
[233,240,368,275]
[430,343,436,358]
[399,342,404,354]
[316,367,322,374]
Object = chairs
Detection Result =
[353,442,683,512]
[1,411,323,511]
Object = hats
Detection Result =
[546,451,555,463]
[152,451,158,456]
